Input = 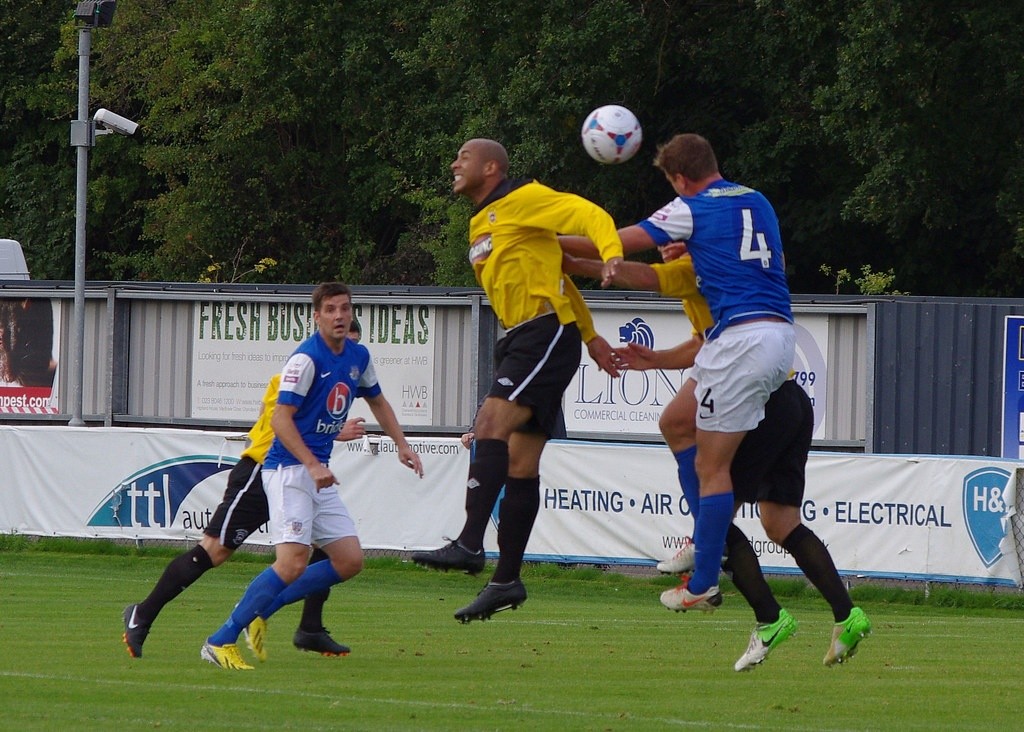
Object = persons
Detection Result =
[200,282,423,669]
[122,317,365,658]
[558,134,871,671]
[411,139,624,623]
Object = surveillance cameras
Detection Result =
[93,108,138,136]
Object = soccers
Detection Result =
[580,102,644,165]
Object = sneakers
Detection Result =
[243,616,268,661]
[823,606,873,667]
[121,604,152,658]
[200,635,255,671]
[454,578,527,622]
[734,608,799,672]
[411,537,485,575]
[293,627,351,656]
[656,537,727,575]
[660,574,722,613]
